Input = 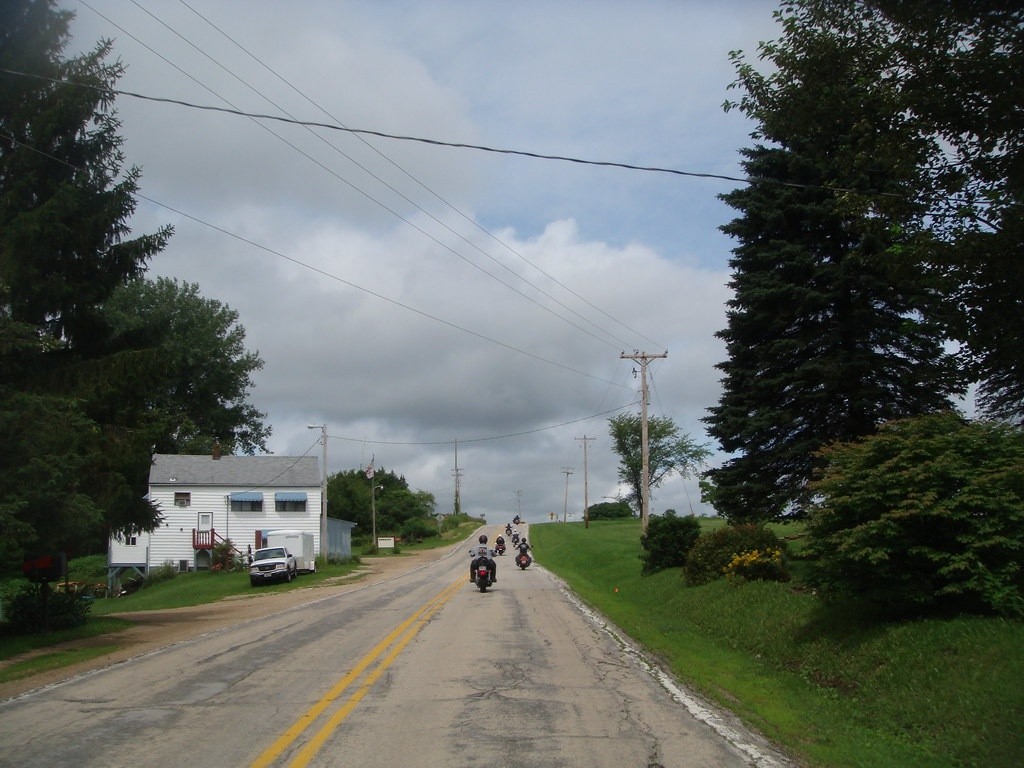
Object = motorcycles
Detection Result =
[507,519,519,545]
[469,550,496,592]
[495,542,507,556]
[512,545,535,569]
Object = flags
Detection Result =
[364,460,374,479]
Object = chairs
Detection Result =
[259,553,268,559]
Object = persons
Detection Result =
[512,528,520,543]
[468,535,497,582]
[514,537,532,566]
[493,534,506,553]
[505,522,512,534]
[512,514,520,523]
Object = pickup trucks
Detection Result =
[249,547,297,586]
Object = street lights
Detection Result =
[371,485,384,544]
[633,368,651,531]
[308,424,328,565]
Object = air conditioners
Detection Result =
[179,560,189,572]
[178,500,186,507]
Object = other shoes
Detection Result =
[492,579,497,582]
[471,578,475,582]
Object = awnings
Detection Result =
[275,492,308,502]
[232,492,263,503]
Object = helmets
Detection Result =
[514,529,516,532]
[479,535,487,544]
[522,538,526,542]
[499,534,502,537]
